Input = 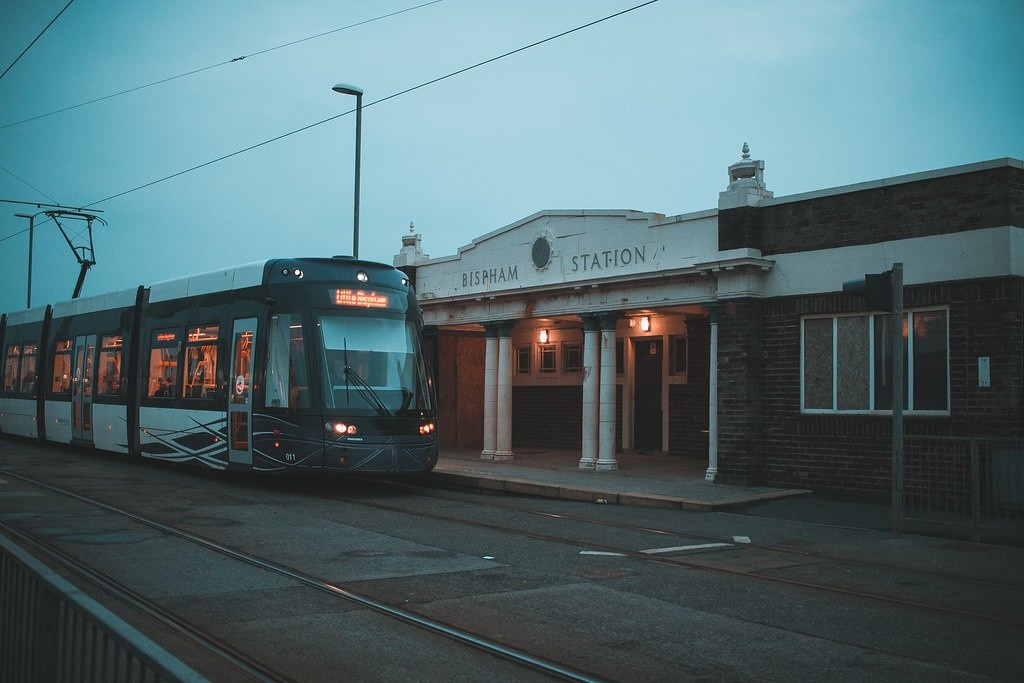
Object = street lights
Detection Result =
[332,80,365,259]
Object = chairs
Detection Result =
[5,378,247,397]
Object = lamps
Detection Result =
[641,316,650,332]
[540,330,548,343]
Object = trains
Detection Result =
[3,253,442,486]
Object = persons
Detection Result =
[60,374,69,391]
[153,375,172,396]
[104,379,119,395]
